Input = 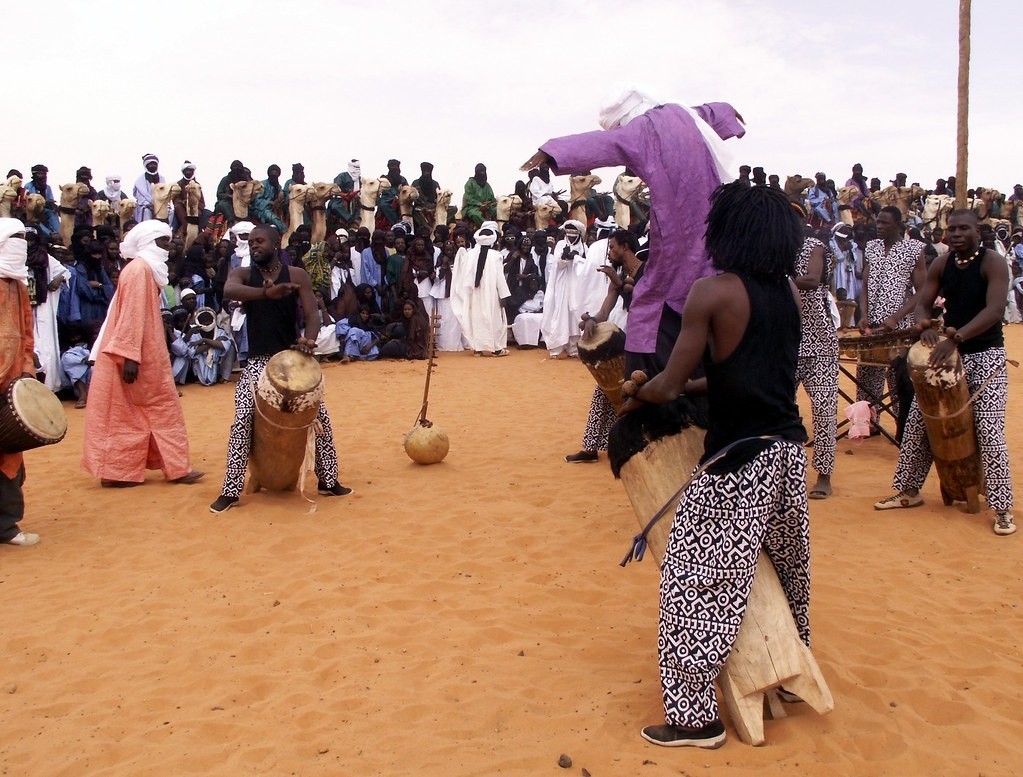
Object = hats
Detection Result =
[161,287,217,332]
[286,224,313,266]
[980,222,1023,248]
[432,214,619,248]
[597,90,743,184]
[740,165,956,195]
[0,217,256,288]
[402,300,420,319]
[356,283,376,326]
[6,152,489,209]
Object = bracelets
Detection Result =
[295,337,318,349]
[914,319,930,333]
[946,327,963,343]
[263,279,275,297]
[579,312,597,331]
[623,277,634,293]
[622,369,648,401]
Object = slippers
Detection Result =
[808,485,832,497]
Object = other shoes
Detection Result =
[474,351,482,356]
[491,349,509,356]
[7,532,39,545]
[75,398,86,407]
[173,471,204,482]
[100,478,141,488]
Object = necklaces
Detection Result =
[955,250,979,265]
[256,261,280,272]
[629,260,640,274]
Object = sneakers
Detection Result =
[874,491,923,509]
[640,719,726,749]
[994,510,1015,534]
[317,480,352,496]
[564,451,597,463]
[209,495,239,512]
[775,686,803,703]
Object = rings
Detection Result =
[530,162,532,164]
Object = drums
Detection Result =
[0,376,69,456]
[576,321,629,412]
[906,333,986,513]
[606,396,837,746]
[248,349,324,492]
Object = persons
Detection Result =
[0,153,1023,440]
[874,209,1016,535]
[209,224,353,514]
[0,218,39,546]
[80,219,206,488]
[618,179,811,749]
[788,200,840,499]
[564,229,647,463]
[518,78,747,382]
